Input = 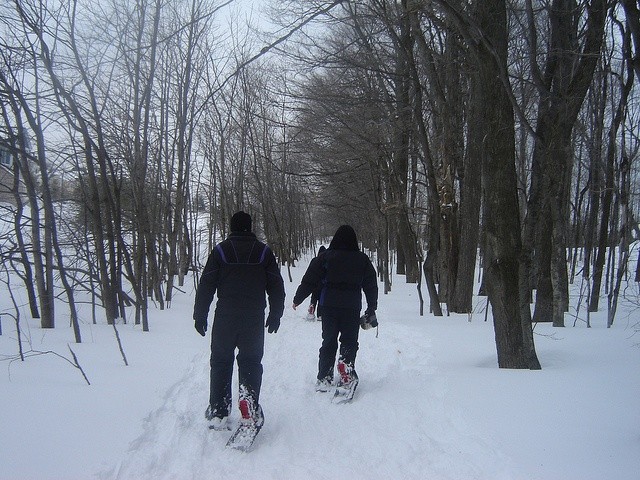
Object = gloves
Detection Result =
[195,319,208,337]
[265,315,280,334]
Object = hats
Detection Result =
[231,212,252,232]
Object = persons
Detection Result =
[308,246,327,321]
[293,225,378,392]
[193,211,286,429]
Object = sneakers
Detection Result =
[337,361,352,386]
[238,393,258,426]
[206,405,228,429]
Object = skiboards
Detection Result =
[310,371,359,402]
[205,403,264,451]
